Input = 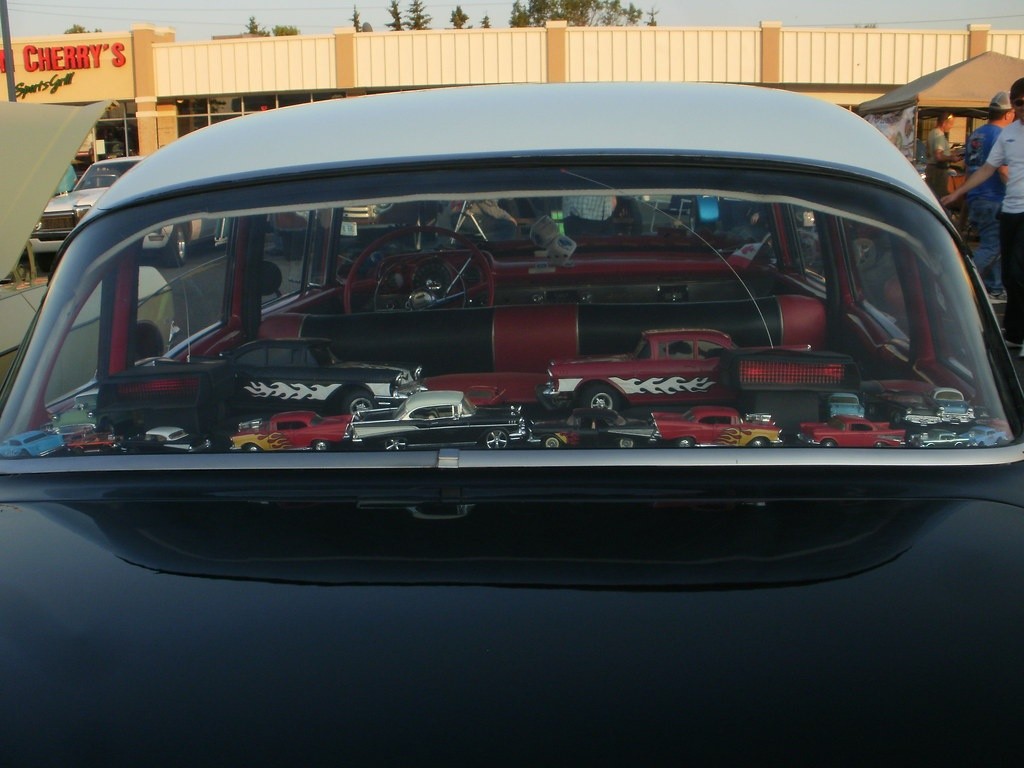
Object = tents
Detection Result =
[859,52,1024,165]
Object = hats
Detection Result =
[990,91,1014,110]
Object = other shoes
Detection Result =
[1006,332,1024,357]
[989,290,1008,304]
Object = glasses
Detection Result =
[944,113,953,121]
[1013,99,1024,106]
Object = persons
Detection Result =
[452,200,515,243]
[964,91,1015,300]
[562,196,617,235]
[940,78,1024,347]
[716,197,766,234]
[927,113,961,198]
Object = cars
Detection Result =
[26,154,229,270]
[274,198,440,265]
[1,82,1022,767]
